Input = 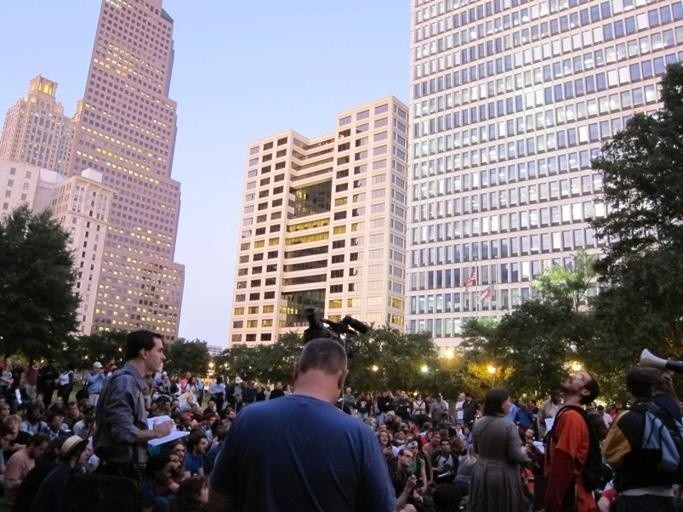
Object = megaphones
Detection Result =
[638,348,683,384]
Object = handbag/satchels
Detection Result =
[75,380,89,403]
[71,469,142,511]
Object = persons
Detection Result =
[93,330,176,485]
[1,353,683,511]
[208,338,397,512]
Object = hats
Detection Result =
[93,361,103,368]
[60,433,90,457]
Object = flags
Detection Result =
[479,286,495,302]
[464,267,475,294]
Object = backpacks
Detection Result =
[544,405,613,493]
[627,405,683,484]
